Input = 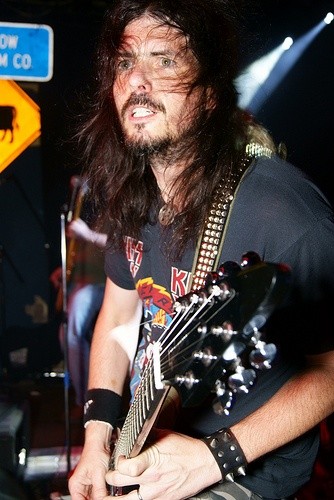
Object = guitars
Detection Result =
[105,251,290,500]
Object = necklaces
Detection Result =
[159,189,181,227]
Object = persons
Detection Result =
[67,0,334,500]
[49,169,109,426]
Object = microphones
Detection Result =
[67,174,81,222]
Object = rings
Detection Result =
[137,490,142,500]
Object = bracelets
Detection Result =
[202,427,249,483]
[82,388,124,428]
